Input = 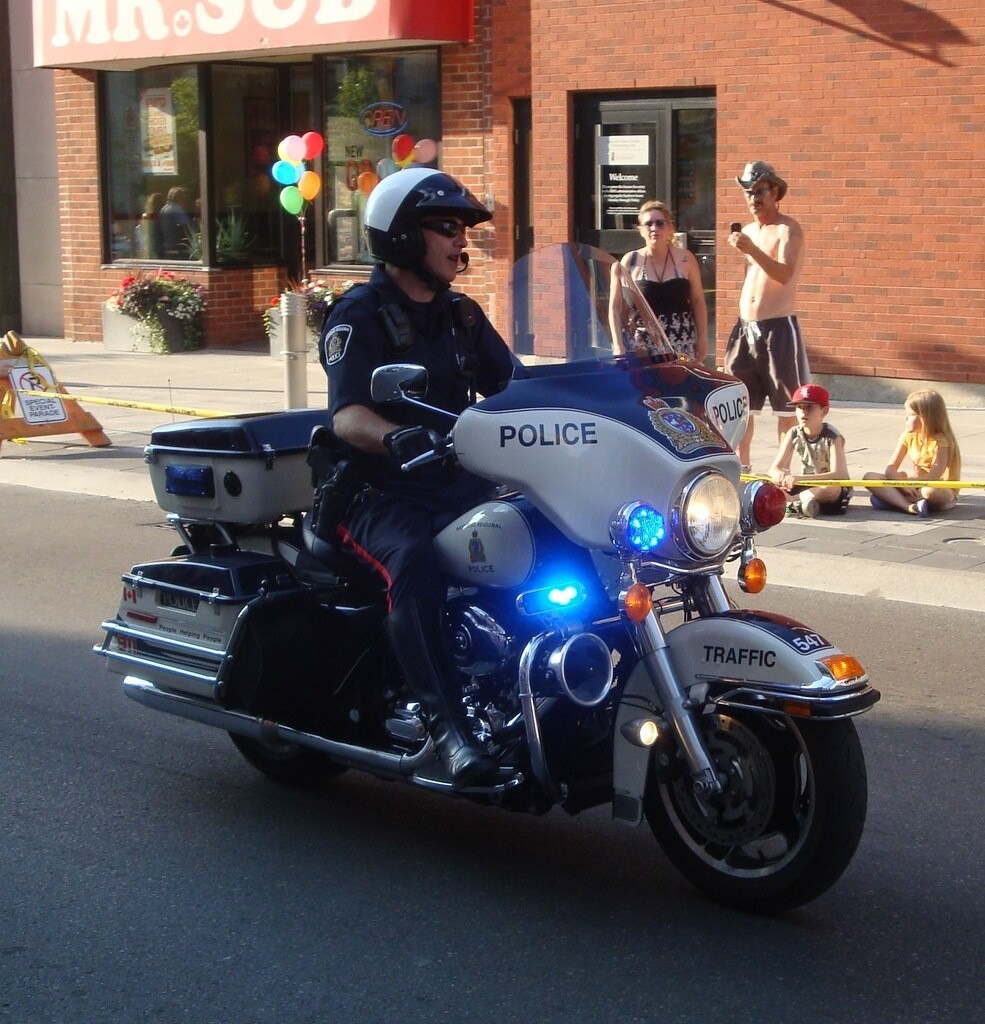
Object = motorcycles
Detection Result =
[85,237,881,921]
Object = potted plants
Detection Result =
[328,66,382,162]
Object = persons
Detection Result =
[767,384,853,518]
[725,161,810,479]
[141,187,200,255]
[609,200,707,363]
[318,166,523,785]
[864,389,962,517]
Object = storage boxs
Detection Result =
[144,408,329,524]
[115,551,300,676]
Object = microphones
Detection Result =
[456,252,469,273]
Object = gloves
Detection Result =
[380,428,452,478]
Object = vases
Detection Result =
[268,308,320,364]
[101,301,185,354]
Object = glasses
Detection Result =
[744,186,773,199]
[421,220,470,238]
[642,221,669,228]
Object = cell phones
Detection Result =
[731,223,741,235]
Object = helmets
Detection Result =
[363,171,493,272]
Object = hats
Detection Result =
[786,385,829,408]
[734,162,786,202]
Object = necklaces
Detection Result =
[648,251,670,283]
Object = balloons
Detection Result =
[278,144,298,166]
[302,131,324,159]
[355,135,436,206]
[283,136,306,161]
[272,161,321,215]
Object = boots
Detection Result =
[385,600,491,786]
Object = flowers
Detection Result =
[108,266,208,354]
[261,277,352,338]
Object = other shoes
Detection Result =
[801,501,819,516]
[912,501,929,517]
[870,494,890,509]
